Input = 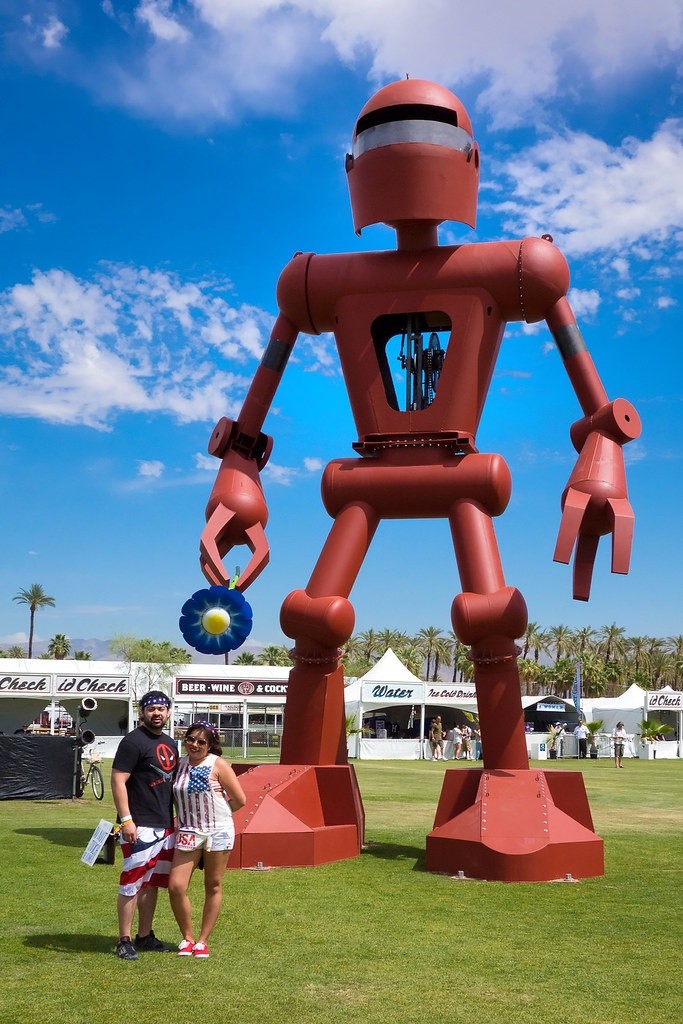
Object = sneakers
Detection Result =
[116,939,138,960]
[177,937,210,958]
[134,930,163,949]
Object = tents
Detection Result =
[344,647,683,760]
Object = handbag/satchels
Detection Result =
[556,729,562,736]
[174,829,211,852]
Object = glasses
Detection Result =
[187,736,209,747]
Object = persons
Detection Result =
[554,722,566,759]
[173,71,639,883]
[429,715,483,761]
[110,690,181,960]
[574,720,591,759]
[169,719,246,958]
[611,721,628,768]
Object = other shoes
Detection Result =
[431,755,448,761]
[465,757,475,760]
[576,755,587,759]
[615,764,623,768]
[453,756,459,760]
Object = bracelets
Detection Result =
[121,814,132,822]
[115,820,133,833]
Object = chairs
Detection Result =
[270,734,281,747]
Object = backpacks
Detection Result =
[467,727,474,738]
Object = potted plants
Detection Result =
[585,720,604,759]
[545,723,567,759]
[346,711,376,761]
[637,719,674,744]
[462,711,482,760]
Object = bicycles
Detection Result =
[80,741,105,800]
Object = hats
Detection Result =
[556,722,561,725]
